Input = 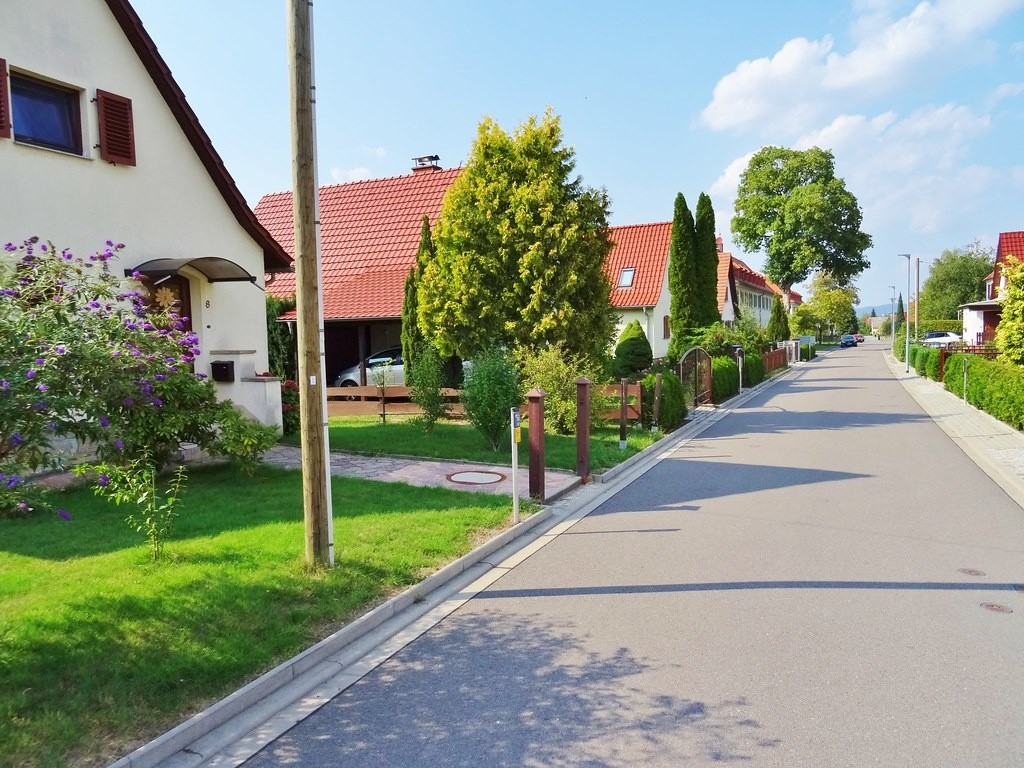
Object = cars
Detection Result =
[853,334,864,343]
[334,345,472,401]
[839,335,858,347]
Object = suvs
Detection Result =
[923,332,963,350]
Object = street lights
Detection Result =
[898,254,911,372]
[888,285,896,346]
